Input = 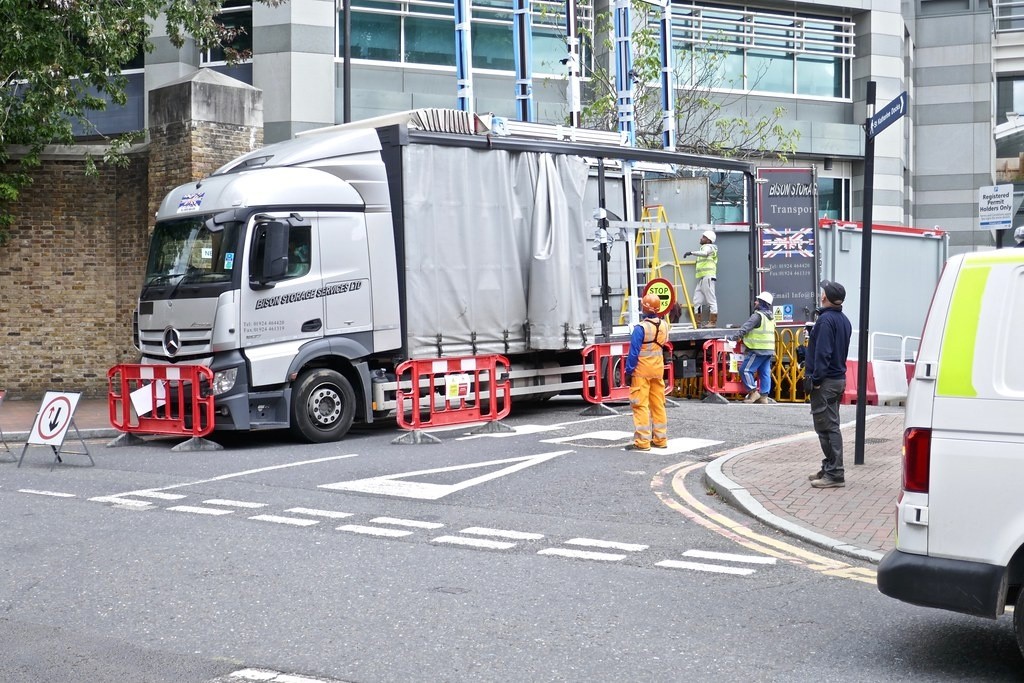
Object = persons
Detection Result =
[733,291,777,406]
[804,279,853,489]
[682,231,718,329]
[624,293,669,451]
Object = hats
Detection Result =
[820,279,847,305]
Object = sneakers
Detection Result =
[754,395,769,405]
[809,469,825,481]
[743,390,762,403]
[811,473,845,487]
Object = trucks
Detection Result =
[131,107,768,440]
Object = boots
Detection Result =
[695,314,701,328]
[704,314,717,328]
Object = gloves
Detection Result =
[625,373,632,386]
[684,252,692,259]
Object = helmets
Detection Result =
[755,291,774,306]
[703,230,717,243]
[641,294,661,314]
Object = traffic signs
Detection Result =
[866,90,909,143]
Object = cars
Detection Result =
[874,221,1024,673]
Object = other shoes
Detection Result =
[650,441,667,448]
[625,444,651,450]
